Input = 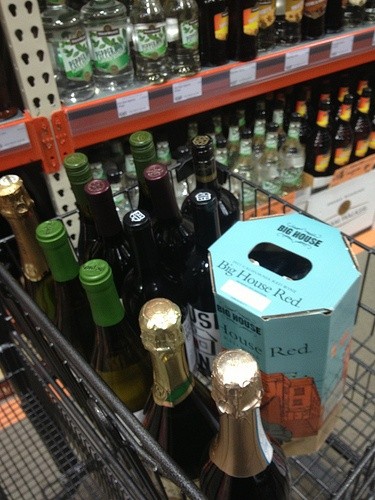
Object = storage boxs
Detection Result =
[244,152,375,235]
[207,214,363,458]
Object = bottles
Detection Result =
[199,348,290,500]
[34,220,95,368]
[120,209,196,376]
[0,175,55,326]
[179,136,239,235]
[79,258,152,453]
[62,154,95,262]
[188,190,220,374]
[140,165,195,278]
[138,298,219,500]
[79,178,133,310]
[42,0,375,222]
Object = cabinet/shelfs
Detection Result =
[0,0,375,250]
